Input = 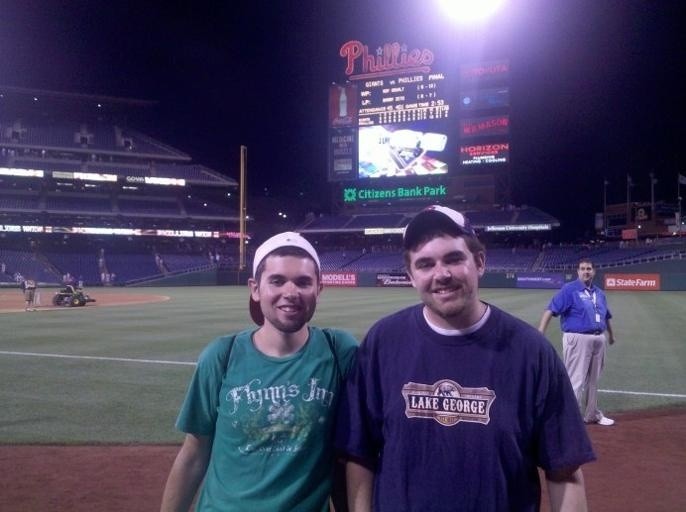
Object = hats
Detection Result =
[248,231,321,327]
[402,205,474,247]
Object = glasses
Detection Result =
[566,330,605,335]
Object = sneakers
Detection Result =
[596,416,615,426]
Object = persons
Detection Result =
[158,232,359,511]
[539,256,616,426]
[340,204,596,512]
[12,271,80,313]
